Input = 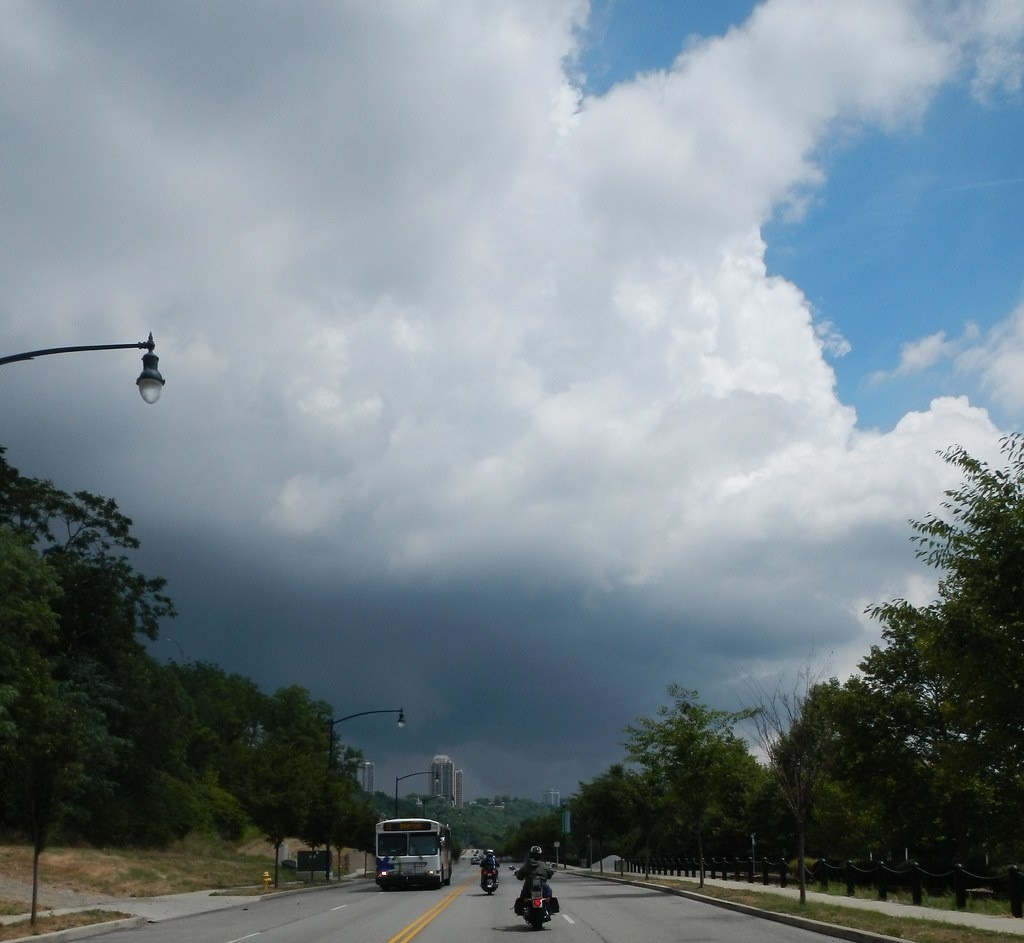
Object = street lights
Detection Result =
[395,771,440,821]
[422,794,455,818]
[326,706,406,882]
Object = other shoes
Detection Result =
[493,879,496,883]
[522,896,528,903]
[546,897,551,904]
[483,877,486,882]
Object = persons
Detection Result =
[479,849,501,884]
[514,845,555,915]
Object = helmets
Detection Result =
[486,848,494,855]
[530,846,542,854]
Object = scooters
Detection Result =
[508,862,560,932]
[480,865,499,895]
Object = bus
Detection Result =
[374,818,453,890]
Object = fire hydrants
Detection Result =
[262,871,273,894]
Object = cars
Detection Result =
[469,854,485,866]
